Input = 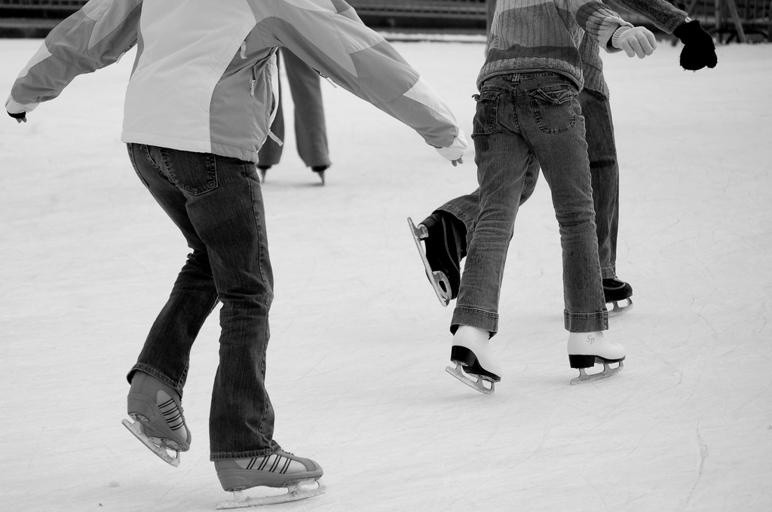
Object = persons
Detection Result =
[253,49,332,188]
[405,0,717,318]
[2,0,469,510]
[438,0,655,395]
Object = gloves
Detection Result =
[674,19,720,73]
[609,22,660,62]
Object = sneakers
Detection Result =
[421,209,466,301]
[447,319,506,384]
[566,328,630,370]
[254,163,330,173]
[210,444,327,495]
[126,363,195,454]
[599,272,635,304]
